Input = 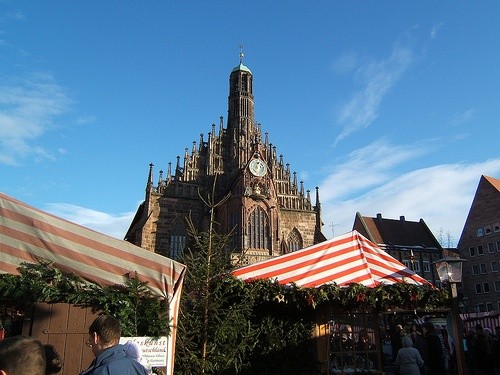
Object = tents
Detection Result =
[220,229,438,290]
[0,192,188,375]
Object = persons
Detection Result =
[438,328,455,370]
[463,324,500,375]
[342,325,353,343]
[396,323,421,340]
[355,329,378,368]
[420,322,443,375]
[395,336,424,375]
[0,335,46,375]
[78,315,149,375]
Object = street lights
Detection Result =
[432,255,467,375]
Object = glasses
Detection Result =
[86,334,95,347]
